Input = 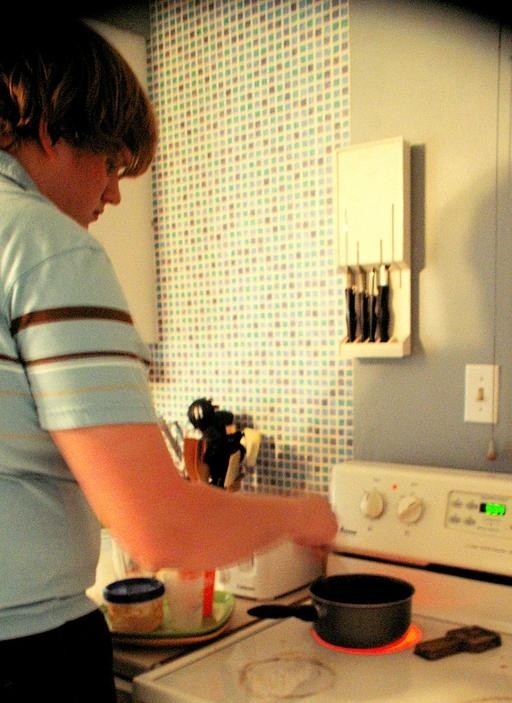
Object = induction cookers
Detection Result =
[130,599,512,700]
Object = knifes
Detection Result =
[340,263,389,344]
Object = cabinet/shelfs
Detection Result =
[84,19,161,345]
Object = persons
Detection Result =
[1,9,338,702]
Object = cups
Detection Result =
[102,566,213,630]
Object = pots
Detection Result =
[244,575,415,649]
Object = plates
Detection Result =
[115,588,234,648]
